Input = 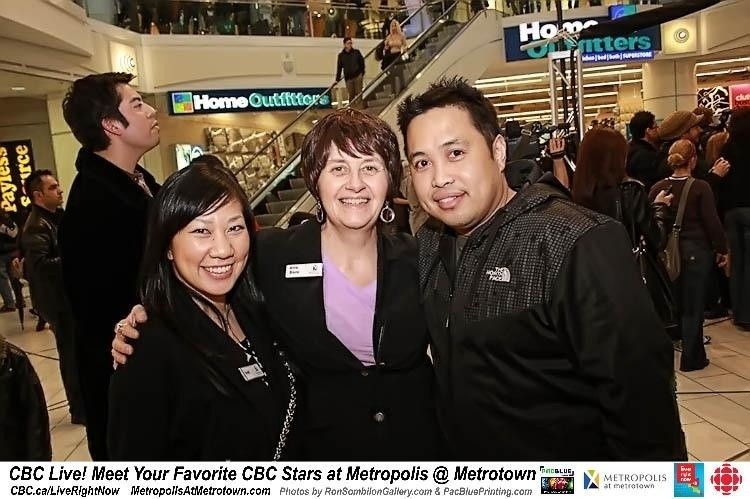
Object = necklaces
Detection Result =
[197,294,274,386]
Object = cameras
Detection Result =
[505,119,578,163]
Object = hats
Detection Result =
[658,108,703,142]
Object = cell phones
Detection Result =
[663,184,674,196]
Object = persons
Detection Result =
[720,106,749,330]
[549,138,570,191]
[22,169,65,354]
[107,165,305,461]
[704,132,730,175]
[0,335,53,463]
[647,111,730,208]
[114,0,411,39]
[398,75,689,462]
[55,81,168,462]
[647,139,728,372]
[625,112,660,184]
[385,20,407,98]
[469,0,658,16]
[335,37,365,110]
[190,154,221,164]
[571,127,674,289]
[0,250,16,311]
[112,110,436,461]
[0,209,28,309]
[693,107,713,129]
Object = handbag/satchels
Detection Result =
[625,189,688,342]
[656,176,695,280]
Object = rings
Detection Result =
[117,322,127,334]
